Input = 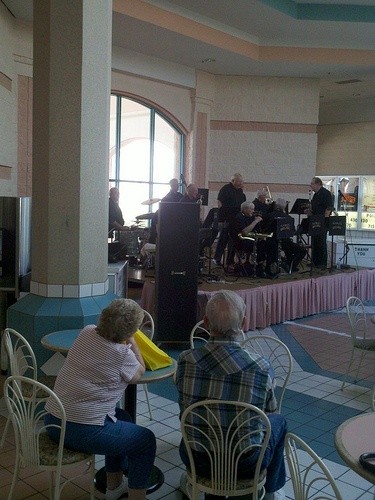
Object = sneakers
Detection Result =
[265,489,285,500]
[180,472,205,500]
[105,474,128,500]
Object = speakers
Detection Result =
[153,202,201,343]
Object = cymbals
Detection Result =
[135,212,155,219]
[141,198,160,206]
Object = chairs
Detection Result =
[180,320,293,500]
[285,433,342,500]
[340,296,375,391]
[139,310,154,421]
[0,328,57,450]
[3,375,95,500]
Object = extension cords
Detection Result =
[207,279,225,284]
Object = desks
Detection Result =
[334,412,375,485]
[41,329,177,425]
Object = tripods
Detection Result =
[293,217,325,276]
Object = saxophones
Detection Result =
[238,231,274,242]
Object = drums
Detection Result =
[117,226,145,257]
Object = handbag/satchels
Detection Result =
[132,329,173,371]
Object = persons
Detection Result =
[173,289,287,500]
[304,178,333,275]
[160,178,183,202]
[177,183,198,202]
[44,298,157,500]
[109,188,140,266]
[212,171,243,266]
[228,185,306,279]
